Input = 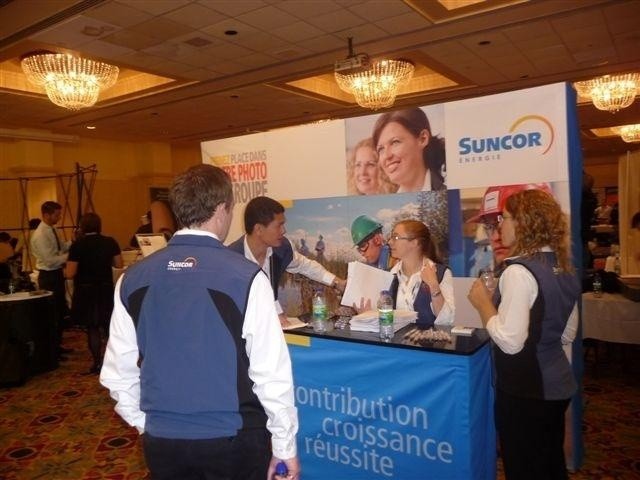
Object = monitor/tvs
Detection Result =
[136,233,166,254]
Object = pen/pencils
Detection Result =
[403,326,452,344]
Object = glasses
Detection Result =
[482,221,498,231]
[390,233,408,240]
[357,235,375,255]
[498,214,513,222]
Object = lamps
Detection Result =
[21,53,120,112]
[334,59,415,112]
[573,73,640,113]
[610,124,640,143]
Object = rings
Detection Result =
[287,474,294,479]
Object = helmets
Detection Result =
[351,215,383,248]
[467,183,551,224]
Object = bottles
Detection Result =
[591,272,603,299]
[377,290,394,342]
[272,462,287,480]
[311,288,328,334]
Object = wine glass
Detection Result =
[8,282,15,296]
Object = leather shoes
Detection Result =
[81,363,101,376]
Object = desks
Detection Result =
[582,292,640,379]
[282,312,497,480]
[0,289,59,378]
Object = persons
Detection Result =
[224,196,347,324]
[130,210,153,247]
[95,161,302,480]
[463,181,553,276]
[315,234,325,259]
[345,138,399,198]
[350,215,399,272]
[371,105,446,198]
[1,217,42,293]
[30,201,75,362]
[68,212,124,368]
[581,170,639,303]
[352,220,456,326]
[466,187,582,480]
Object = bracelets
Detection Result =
[333,280,338,288]
[432,291,442,297]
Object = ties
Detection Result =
[53,228,61,251]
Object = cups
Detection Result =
[480,271,497,292]
